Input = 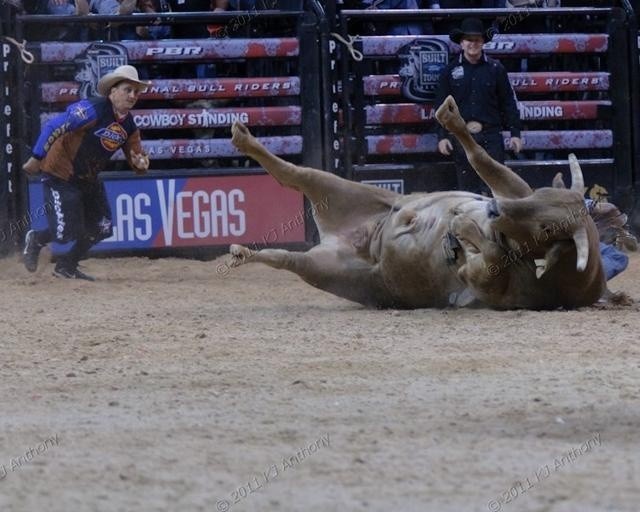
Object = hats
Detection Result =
[96,65,153,96]
[450,18,492,44]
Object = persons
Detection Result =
[45,1,607,166]
[432,35,523,197]
[21,66,153,282]
[585,198,629,281]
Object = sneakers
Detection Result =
[52,267,95,282]
[23,229,48,273]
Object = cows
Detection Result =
[229,95,605,311]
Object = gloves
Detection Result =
[509,137,521,153]
[131,153,150,172]
[22,156,40,176]
[438,138,453,156]
